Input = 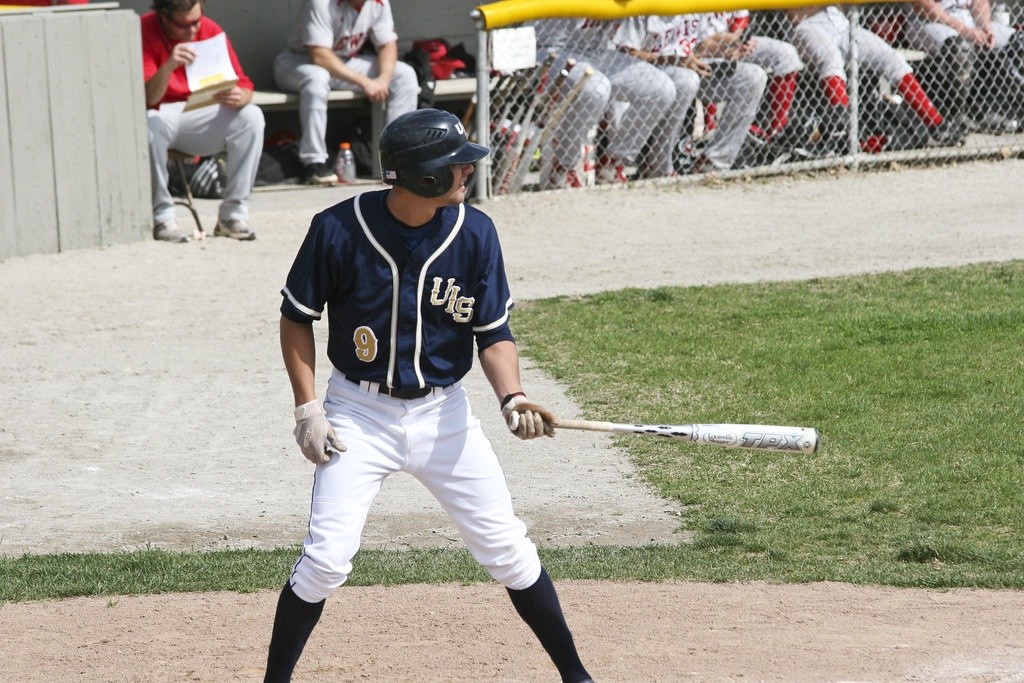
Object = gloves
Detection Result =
[293,398,347,465]
[502,392,559,441]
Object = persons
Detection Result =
[140,0,266,242]
[263,107,594,683]
[274,0,418,183]
[522,1,1024,190]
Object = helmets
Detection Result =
[378,110,490,197]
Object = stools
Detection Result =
[165,150,204,234]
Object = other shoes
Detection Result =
[537,154,582,190]
[303,163,340,185]
[592,161,628,184]
[690,157,724,189]
[640,162,680,179]
[152,221,184,241]
[212,219,257,241]
[747,111,1024,160]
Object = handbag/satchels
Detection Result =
[164,115,375,199]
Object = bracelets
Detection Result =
[501,392,526,410]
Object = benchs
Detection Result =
[247,77,475,179]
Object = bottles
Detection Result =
[335,142,356,184]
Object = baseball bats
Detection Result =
[509,411,819,455]
[461,54,594,202]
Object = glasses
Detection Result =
[167,13,202,28]
